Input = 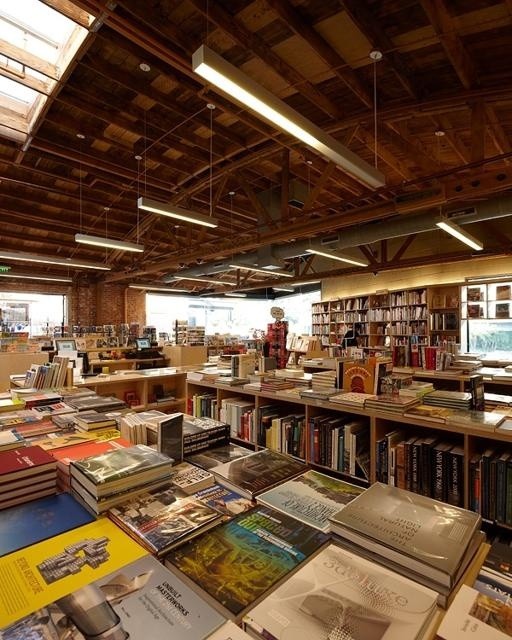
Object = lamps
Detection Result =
[137,61,219,229]
[192,1,387,190]
[304,159,369,268]
[74,133,146,252]
[434,129,484,251]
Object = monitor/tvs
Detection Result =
[56,340,77,352]
[135,338,152,349]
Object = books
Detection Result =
[313,288,430,354]
[186,331,511,394]
[24,354,69,389]
[1,385,511,640]
